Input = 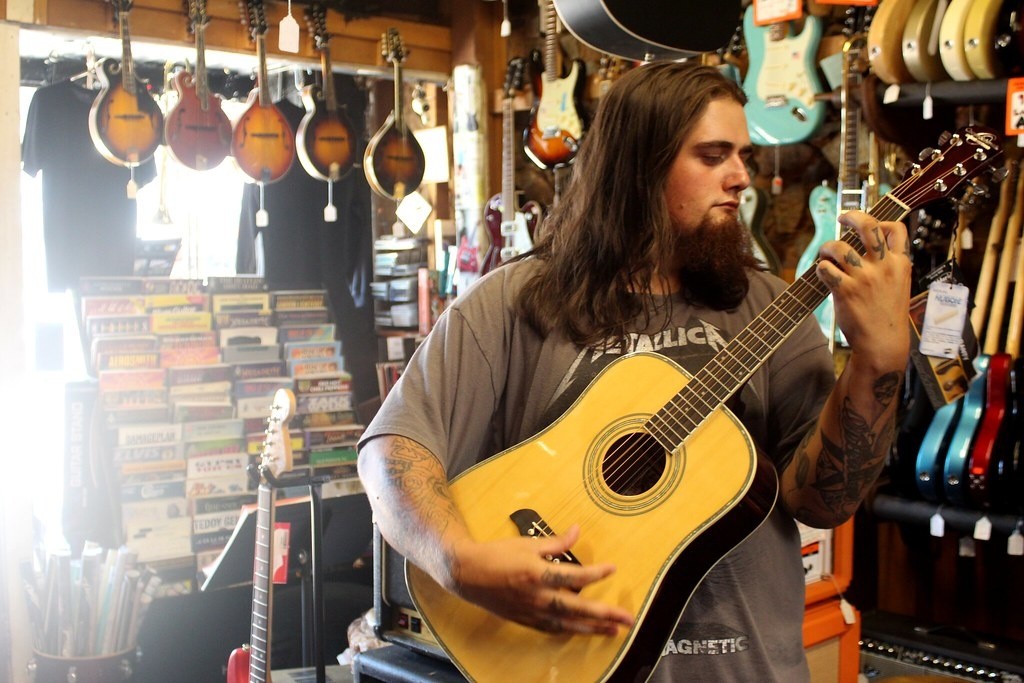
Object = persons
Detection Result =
[357,62,912,683]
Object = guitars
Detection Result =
[296,0,360,181]
[363,27,426,199]
[230,0,296,182]
[406,127,998,683]
[746,102,1024,511]
[554,0,750,63]
[523,0,590,166]
[164,1,232,168]
[226,388,297,683]
[89,0,165,164]
[814,0,1023,85]
[741,0,823,144]
[481,55,549,274]
[796,6,899,344]
